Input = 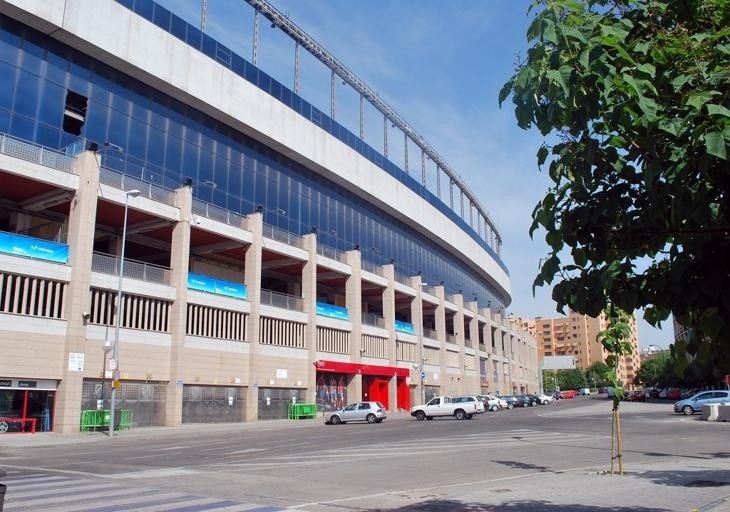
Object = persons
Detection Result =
[554,387,561,408]
[316,373,347,410]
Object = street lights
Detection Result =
[110,189,140,437]
[649,345,664,374]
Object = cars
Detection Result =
[324,401,387,424]
[622,388,701,402]
[599,387,607,394]
[559,388,590,399]
[410,394,553,420]
[674,390,730,415]
